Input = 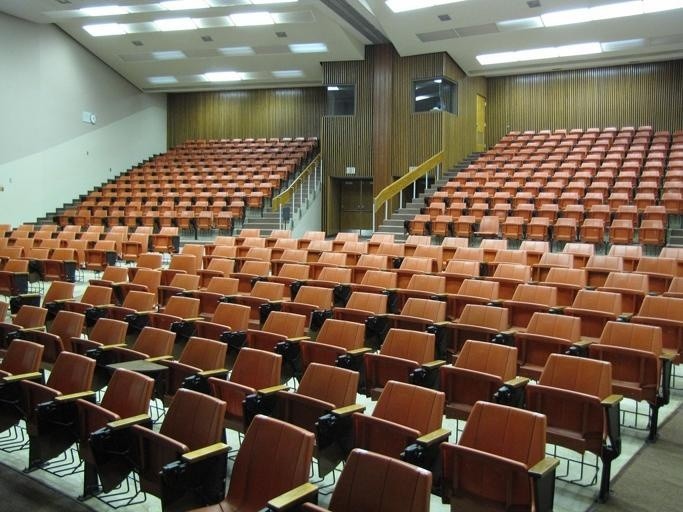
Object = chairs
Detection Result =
[2,120,682,509]
